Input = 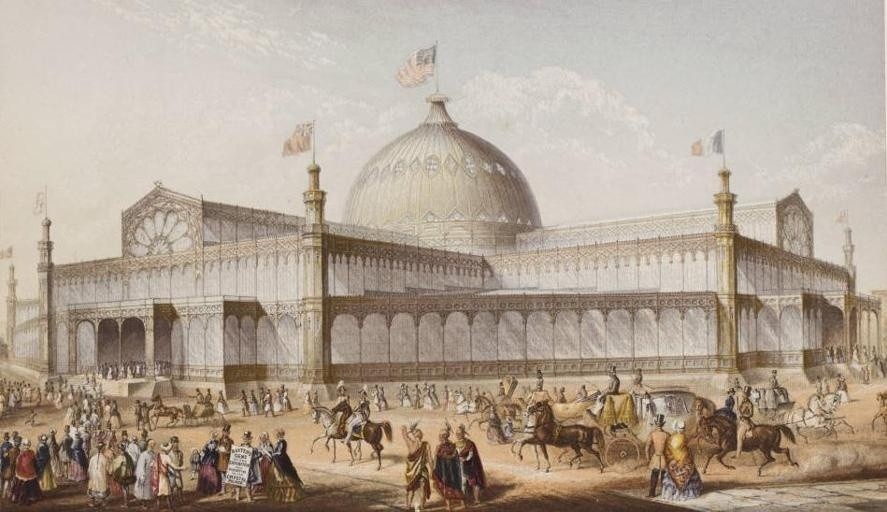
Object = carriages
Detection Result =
[509,387,716,473]
[733,386,856,447]
[147,393,225,432]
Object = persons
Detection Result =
[0,343,887,512]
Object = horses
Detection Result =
[325,398,392,471]
[309,406,362,467]
[820,393,856,440]
[698,415,799,476]
[468,394,523,432]
[779,393,833,446]
[511,398,569,470]
[517,398,606,474]
[687,396,721,455]
[150,394,183,430]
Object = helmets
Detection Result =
[338,386,366,395]
[413,428,465,438]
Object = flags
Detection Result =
[834,208,850,225]
[282,121,314,156]
[394,45,436,88]
[31,190,47,215]
[690,129,725,156]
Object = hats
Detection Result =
[209,425,285,441]
[608,366,617,373]
[654,414,686,431]
[4,425,70,448]
[121,430,179,451]
[727,386,752,396]
